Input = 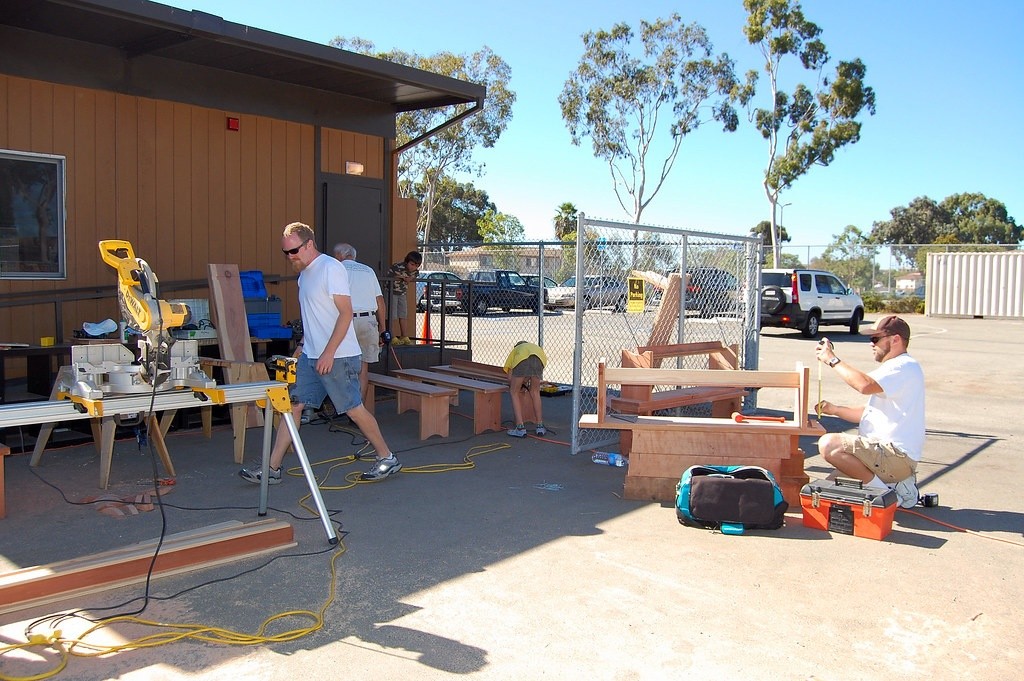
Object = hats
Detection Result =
[870,315,910,340]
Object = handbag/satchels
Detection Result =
[674,464,790,536]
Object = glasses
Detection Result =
[282,238,311,255]
[870,333,898,345]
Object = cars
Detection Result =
[501,274,628,312]
[909,286,925,301]
[415,270,465,313]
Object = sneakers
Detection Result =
[894,471,919,509]
[881,489,902,507]
[507,428,527,438]
[239,465,282,484]
[536,425,546,436]
[361,450,403,481]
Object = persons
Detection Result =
[384,251,422,346]
[333,244,385,428]
[240,222,403,482]
[816,316,925,507]
[503,342,546,438]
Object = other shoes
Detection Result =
[401,336,413,345]
[390,337,404,345]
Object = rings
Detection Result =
[324,369,327,370]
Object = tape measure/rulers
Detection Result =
[818,340,834,419]
[609,413,638,423]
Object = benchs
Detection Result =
[579,341,827,457]
[434,365,546,425]
[397,370,508,433]
[365,371,457,441]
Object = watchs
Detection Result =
[830,357,840,367]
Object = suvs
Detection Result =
[737,269,865,338]
[651,266,746,313]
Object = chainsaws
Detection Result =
[60,239,217,397]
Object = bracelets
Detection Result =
[297,343,304,347]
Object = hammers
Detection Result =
[731,412,785,424]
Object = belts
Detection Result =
[353,311,375,317]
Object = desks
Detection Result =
[73,337,272,362]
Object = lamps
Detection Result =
[346,161,363,176]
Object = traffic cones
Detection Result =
[421,311,434,347]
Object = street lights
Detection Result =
[775,202,792,260]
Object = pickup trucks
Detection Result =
[421,269,539,317]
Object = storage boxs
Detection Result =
[240,267,293,339]
[168,293,218,340]
[799,476,898,542]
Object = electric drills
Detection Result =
[378,331,392,362]
